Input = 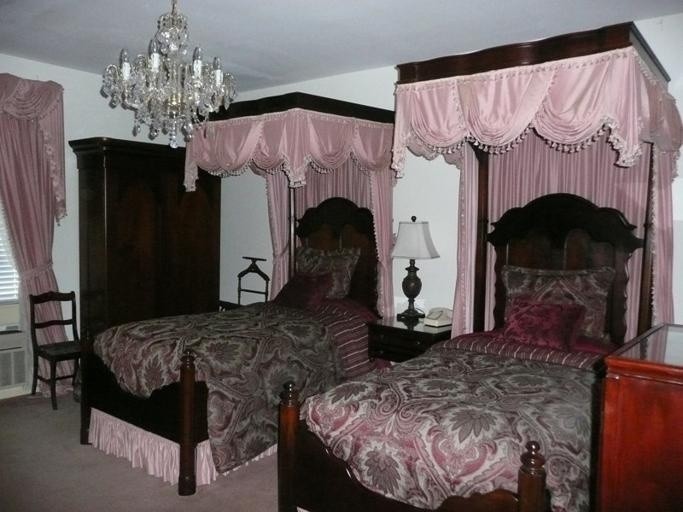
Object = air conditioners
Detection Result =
[0,328,27,390]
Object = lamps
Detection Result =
[389,212,441,321]
[99,1,239,148]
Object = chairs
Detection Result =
[27,289,84,411]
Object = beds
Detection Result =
[74,196,373,495]
[279,189,641,511]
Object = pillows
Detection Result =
[496,262,617,351]
[291,244,359,298]
[498,293,587,353]
[273,272,335,312]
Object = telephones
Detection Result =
[424,307,453,327]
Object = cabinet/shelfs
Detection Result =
[595,322,683,512]
[66,136,223,359]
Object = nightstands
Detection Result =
[365,314,455,364]
